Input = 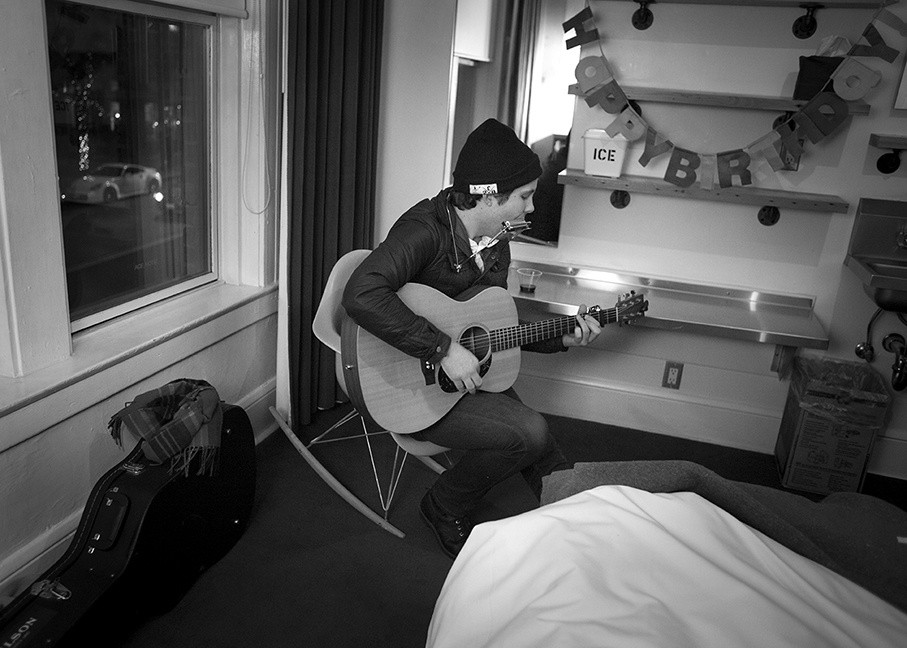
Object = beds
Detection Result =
[421,460,906,648]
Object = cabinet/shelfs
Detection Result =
[557,75,853,214]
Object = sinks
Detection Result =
[844,255,907,309]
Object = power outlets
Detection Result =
[662,359,684,390]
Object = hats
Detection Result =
[453,118,543,195]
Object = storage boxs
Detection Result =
[581,127,634,180]
[774,355,893,499]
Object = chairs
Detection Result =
[269,248,453,538]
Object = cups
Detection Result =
[516,267,543,292]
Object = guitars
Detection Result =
[340,283,649,435]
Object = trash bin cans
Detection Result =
[772,357,893,496]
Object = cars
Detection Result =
[67,163,162,206]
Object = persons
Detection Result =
[335,116,601,557]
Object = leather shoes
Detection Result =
[418,489,473,560]
